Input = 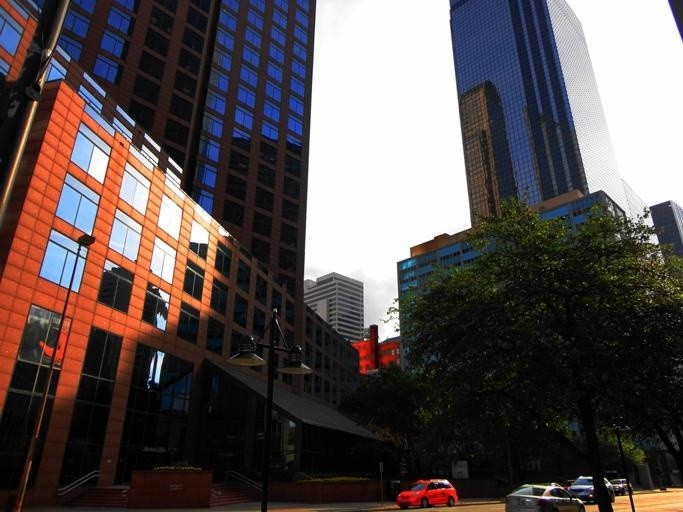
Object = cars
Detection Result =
[395,479,459,508]
[505,476,632,512]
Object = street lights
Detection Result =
[13,235,96,512]
[226,306,314,511]
